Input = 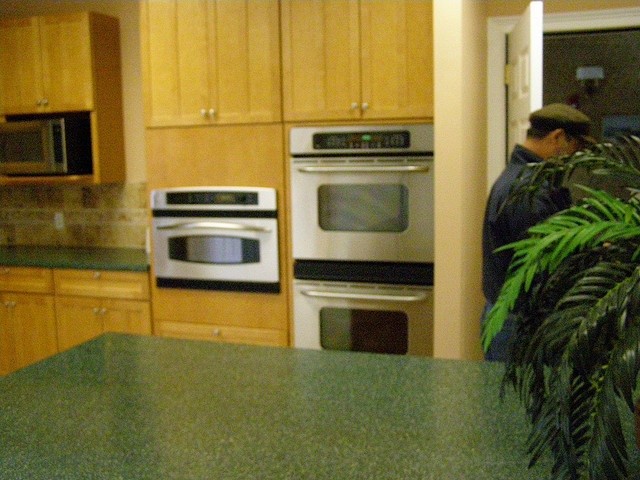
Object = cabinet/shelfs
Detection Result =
[1,13,121,111]
[138,0,289,130]
[0,264,156,375]
[280,1,434,122]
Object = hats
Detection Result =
[529,102,597,145]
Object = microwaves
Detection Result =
[149,184,281,295]
[0,119,70,176]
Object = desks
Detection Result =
[2,331,640,480]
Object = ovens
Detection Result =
[289,126,436,288]
[291,280,434,358]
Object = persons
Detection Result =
[481,102,596,364]
